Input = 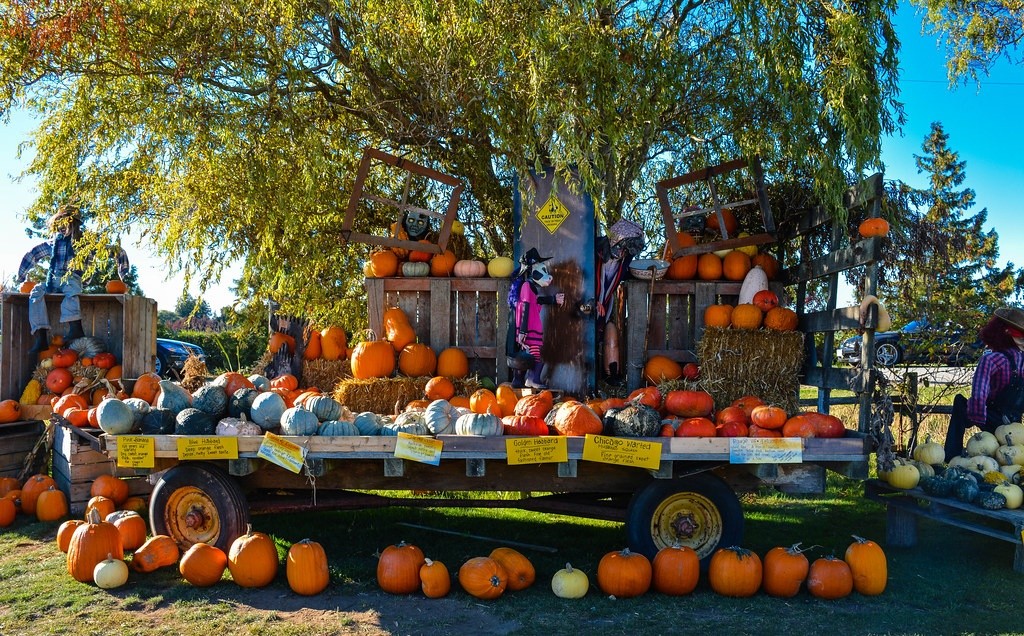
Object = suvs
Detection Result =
[834,318,983,366]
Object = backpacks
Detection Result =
[986,376,1024,429]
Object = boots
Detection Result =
[64,321,85,341]
[512,361,550,389]
[28,329,49,355]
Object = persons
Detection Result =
[401,204,430,239]
[966,305,1024,434]
[15,205,133,354]
[679,203,708,236]
[504,244,567,390]
[595,215,650,380]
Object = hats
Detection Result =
[994,307,1024,330]
[607,217,643,247]
[516,247,554,280]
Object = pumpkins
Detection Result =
[764,541,810,597]
[662,210,776,281]
[598,548,652,597]
[0,215,514,435]
[846,534,889,596]
[502,355,846,438]
[861,217,890,237]
[1,469,66,526]
[57,475,328,595]
[876,420,1023,508]
[709,545,762,595]
[808,550,854,597]
[458,556,506,597]
[550,562,589,599]
[654,536,700,595]
[491,546,536,589]
[377,539,423,593]
[705,290,797,331]
[418,557,451,599]
